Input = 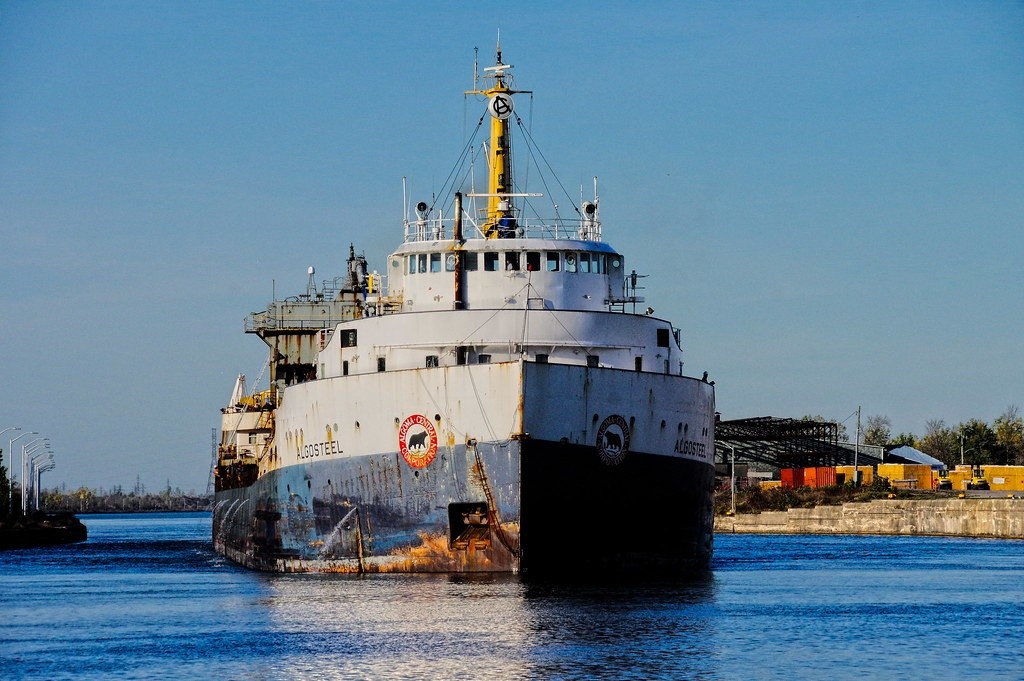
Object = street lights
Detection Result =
[8,431,38,516]
[715,440,734,509]
[21,437,56,516]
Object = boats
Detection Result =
[213,25,715,586]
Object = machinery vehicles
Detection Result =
[937,469,953,492]
[966,464,990,490]
